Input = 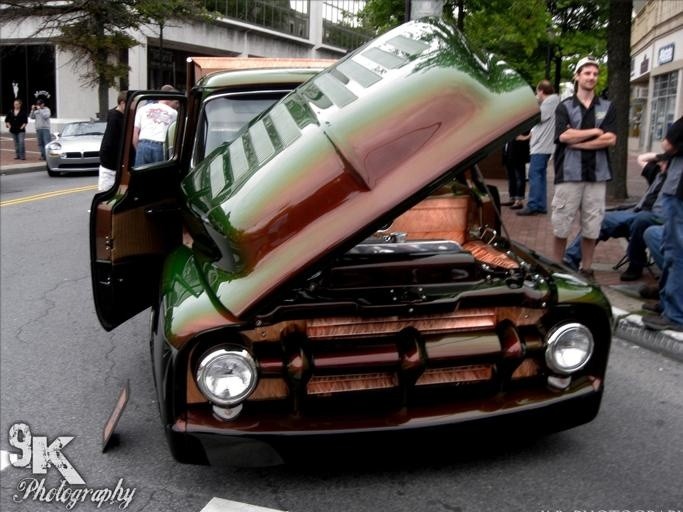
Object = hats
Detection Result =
[572,56,599,91]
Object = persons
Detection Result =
[516,81,560,217]
[5,100,27,159]
[501,129,533,209]
[561,148,669,280]
[550,54,616,280]
[132,84,179,167]
[639,116,683,333]
[638,226,668,299]
[97,90,126,190]
[29,99,52,160]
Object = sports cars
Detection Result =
[41,120,107,178]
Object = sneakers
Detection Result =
[639,284,660,299]
[641,314,682,332]
[620,262,642,282]
[641,302,660,314]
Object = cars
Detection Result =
[88,16,615,464]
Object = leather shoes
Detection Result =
[500,200,547,215]
